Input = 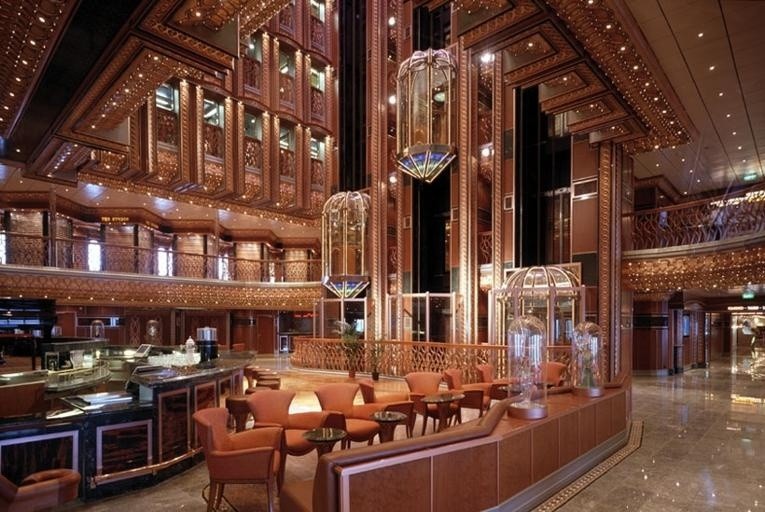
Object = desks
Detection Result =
[368,410,408,444]
[419,392,466,433]
[302,427,348,464]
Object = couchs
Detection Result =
[278,372,632,512]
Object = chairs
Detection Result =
[532,362,567,389]
[444,368,494,424]
[0,469,82,512]
[358,380,418,439]
[403,371,465,438]
[192,406,284,512]
[245,389,337,498]
[475,364,520,412]
[311,382,390,449]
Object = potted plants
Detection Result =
[368,333,386,381]
[343,322,362,379]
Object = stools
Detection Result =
[225,364,281,433]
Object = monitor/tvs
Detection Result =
[134,344,152,358]
[125,365,163,393]
